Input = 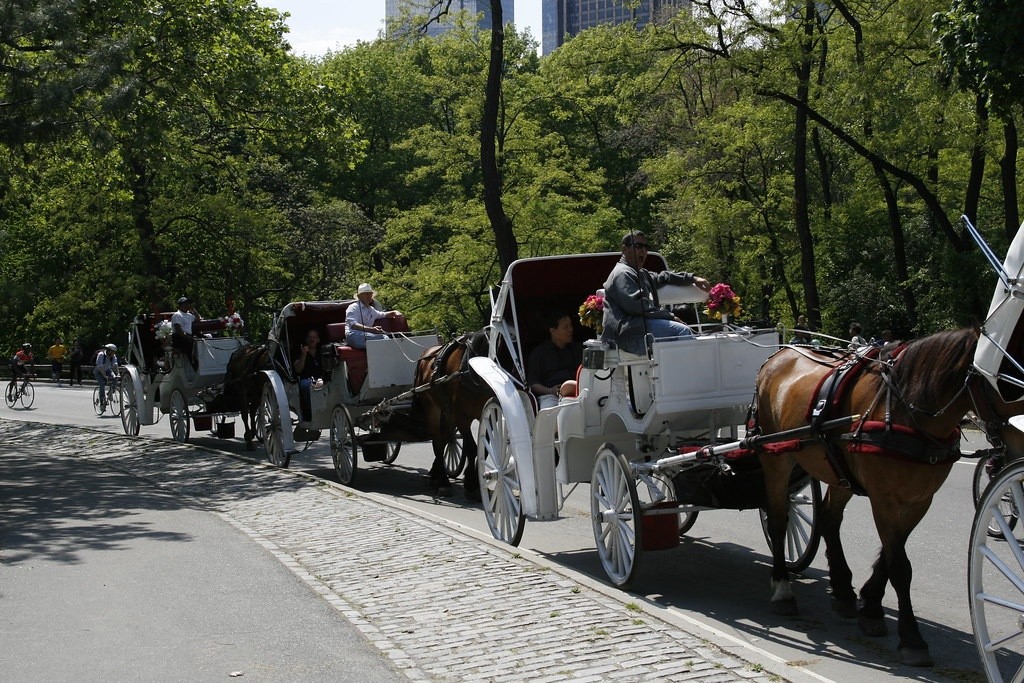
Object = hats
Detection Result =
[177,296,189,304]
[22,343,32,347]
[357,283,374,296]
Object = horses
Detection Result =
[413,328,526,500]
[748,327,1024,666]
[225,344,274,452]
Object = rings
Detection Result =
[557,388,559,391]
[557,392,559,395]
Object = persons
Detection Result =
[70,340,83,386]
[7,343,34,402]
[48,337,68,387]
[171,297,205,372]
[602,230,711,358]
[527,309,585,439]
[345,283,397,349]
[788,313,893,350]
[93,344,119,412]
[294,329,326,421]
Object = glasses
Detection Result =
[624,242,651,250]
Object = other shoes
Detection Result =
[52,374,55,380]
[57,382,61,387]
[19,387,27,395]
[106,392,114,401]
[7,394,13,402]
[101,402,106,411]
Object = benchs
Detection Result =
[327,314,411,362]
[595,283,708,366]
[160,319,221,352]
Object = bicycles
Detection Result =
[5,373,37,409]
[958,410,1023,538]
[92,376,124,416]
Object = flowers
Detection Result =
[578,296,604,334]
[219,312,244,336]
[149,320,174,344]
[701,283,744,319]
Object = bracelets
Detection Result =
[550,388,552,393]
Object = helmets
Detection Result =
[104,343,117,352]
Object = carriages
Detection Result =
[958,209,1024,682]
[463,249,987,668]
[116,307,273,452]
[257,298,513,497]
